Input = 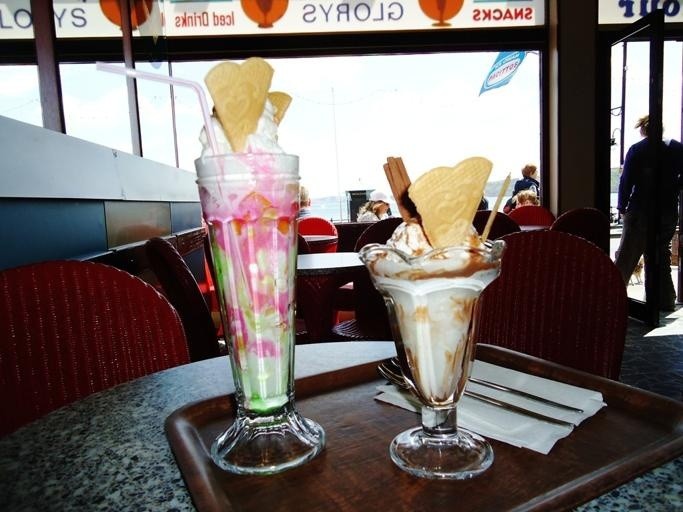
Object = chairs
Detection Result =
[506,205,553,230]
[1,260,191,438]
[481,230,628,381]
[298,215,338,253]
[354,217,404,253]
[552,208,611,247]
[144,236,221,362]
[472,210,521,241]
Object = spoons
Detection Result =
[375,361,577,430]
[391,356,582,413]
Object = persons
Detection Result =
[615,115,678,311]
[357,191,389,222]
[515,164,540,195]
[478,192,487,210]
[513,190,538,209]
[300,186,312,218]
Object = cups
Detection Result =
[195,155,325,475]
[359,239,505,480]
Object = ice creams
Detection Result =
[193,59,326,477]
[359,157,511,481]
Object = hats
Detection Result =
[370,191,392,204]
